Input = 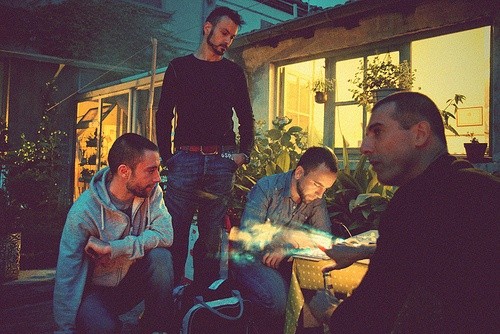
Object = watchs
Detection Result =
[241,153,250,164]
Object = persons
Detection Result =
[155,6,254,285]
[51,133,175,334]
[310,91,500,334]
[235,148,340,334]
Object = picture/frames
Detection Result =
[456,106,483,127]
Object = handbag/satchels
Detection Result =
[160,277,264,334]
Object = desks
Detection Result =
[283,230,379,334]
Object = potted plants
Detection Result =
[81,128,107,179]
[464,138,487,162]
[312,78,334,104]
[348,54,417,111]
[0,116,69,282]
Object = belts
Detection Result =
[173,143,236,156]
